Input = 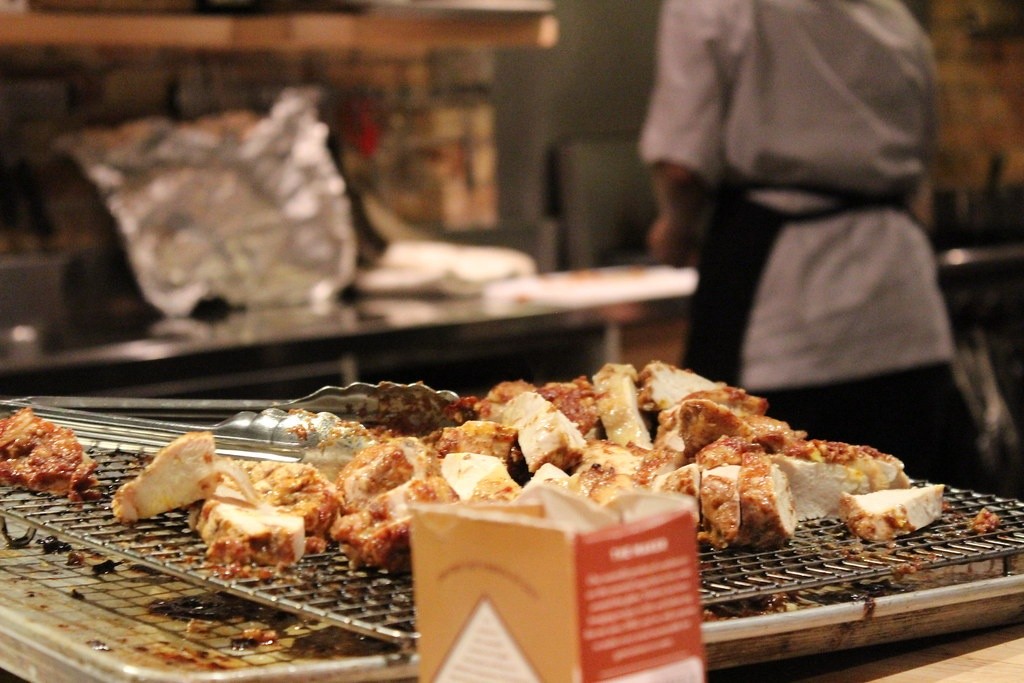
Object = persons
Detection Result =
[639,0,984,493]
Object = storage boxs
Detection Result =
[408,503,705,683]
